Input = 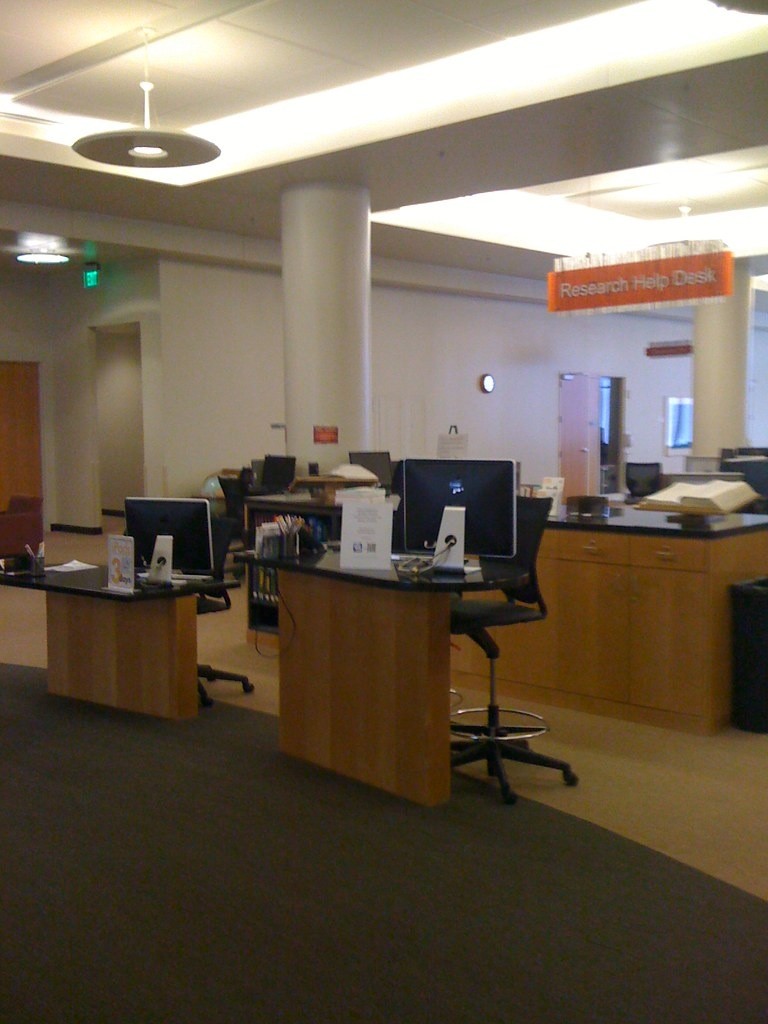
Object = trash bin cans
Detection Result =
[729,577,768,733]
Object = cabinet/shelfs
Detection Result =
[447,527,562,709]
[563,527,767,737]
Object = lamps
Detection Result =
[72,26,220,167]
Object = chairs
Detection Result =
[622,461,660,505]
[449,495,581,799]
[176,517,255,705]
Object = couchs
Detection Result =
[1,493,45,560]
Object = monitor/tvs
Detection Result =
[261,455,296,495]
[402,458,516,575]
[349,451,393,488]
[123,497,214,586]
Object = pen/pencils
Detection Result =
[271,515,304,560]
[22,542,45,574]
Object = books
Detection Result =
[250,517,328,604]
[632,479,760,515]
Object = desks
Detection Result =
[242,493,342,541]
[295,475,377,493]
[233,545,529,808]
[0,561,239,720]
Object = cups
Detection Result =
[281,536,296,557]
[30,557,45,576]
